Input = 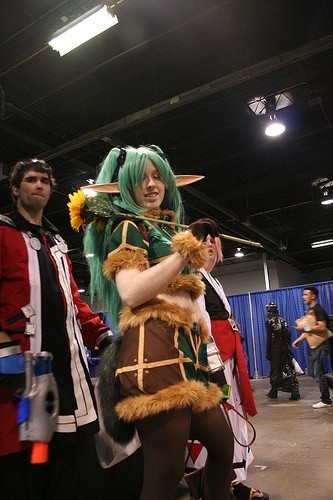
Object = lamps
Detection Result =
[234,248,244,257]
[46,2,119,58]
[321,187,333,205]
[265,95,286,137]
[311,239,333,249]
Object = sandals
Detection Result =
[232,482,269,500]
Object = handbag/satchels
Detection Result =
[184,463,237,500]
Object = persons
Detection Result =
[0,158,125,500]
[75,143,239,500]
[263,302,302,401]
[174,217,272,500]
[291,286,333,408]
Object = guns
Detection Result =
[10,350,61,464]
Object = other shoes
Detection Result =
[313,401,331,408]
[289,393,300,400]
[266,391,277,399]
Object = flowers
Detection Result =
[66,189,263,248]
